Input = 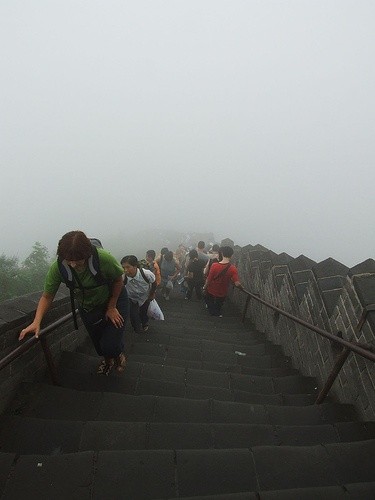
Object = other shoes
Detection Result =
[217,313,223,318]
[183,296,187,300]
[141,324,148,331]
[165,295,169,300]
[188,298,192,301]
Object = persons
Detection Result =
[145,241,245,317]
[121,255,157,334]
[18,231,129,377]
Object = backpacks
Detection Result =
[124,259,151,303]
[57,237,102,330]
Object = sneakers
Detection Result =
[114,350,127,373]
[96,357,113,378]
